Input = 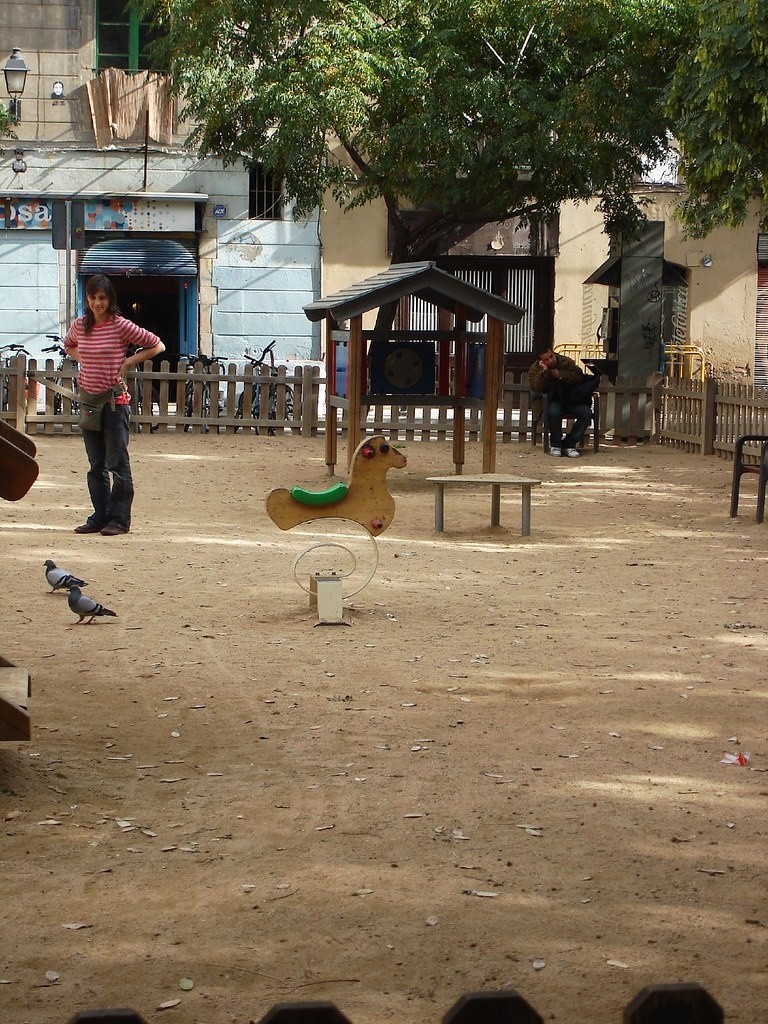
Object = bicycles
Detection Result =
[1,335,161,432]
[179,340,298,434]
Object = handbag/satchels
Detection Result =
[553,374,599,403]
[78,383,125,432]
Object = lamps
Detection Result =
[0,47,31,98]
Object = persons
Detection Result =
[63,274,166,535]
[528,344,592,457]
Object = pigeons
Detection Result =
[42,559,119,625]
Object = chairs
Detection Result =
[531,390,599,455]
[730,434,768,525]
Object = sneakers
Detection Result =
[550,447,562,456]
[563,448,579,457]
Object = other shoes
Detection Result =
[73,524,99,533]
[100,525,126,535]
